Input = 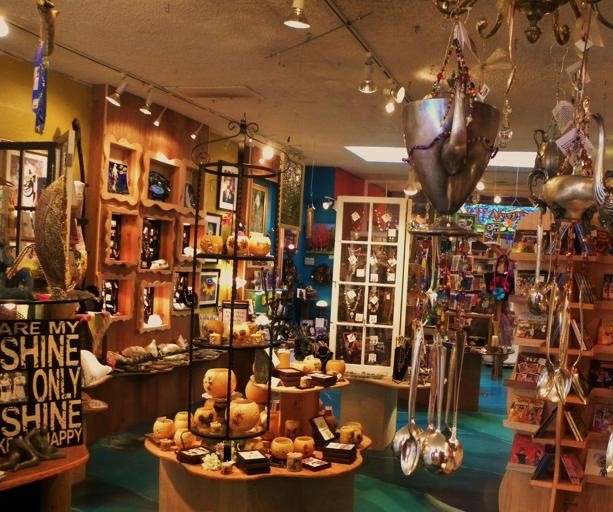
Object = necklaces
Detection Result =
[341,204,398,360]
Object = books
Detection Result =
[509,229,612,486]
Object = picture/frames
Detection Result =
[2,151,49,250]
[217,159,238,213]
[196,268,221,307]
[251,182,268,239]
[205,211,223,237]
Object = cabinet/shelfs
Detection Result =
[3,287,96,512]
[145,434,371,511]
[82,82,280,443]
[343,367,447,459]
[328,196,412,380]
[189,113,290,440]
[498,251,612,512]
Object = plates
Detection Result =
[149,170,172,201]
[186,182,197,208]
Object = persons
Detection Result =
[223,177,234,203]
[142,287,151,321]
[111,162,118,192]
[251,189,262,233]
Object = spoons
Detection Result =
[394,325,465,477]
[527,222,589,405]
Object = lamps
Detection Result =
[0,12,297,164]
[282,0,406,113]
